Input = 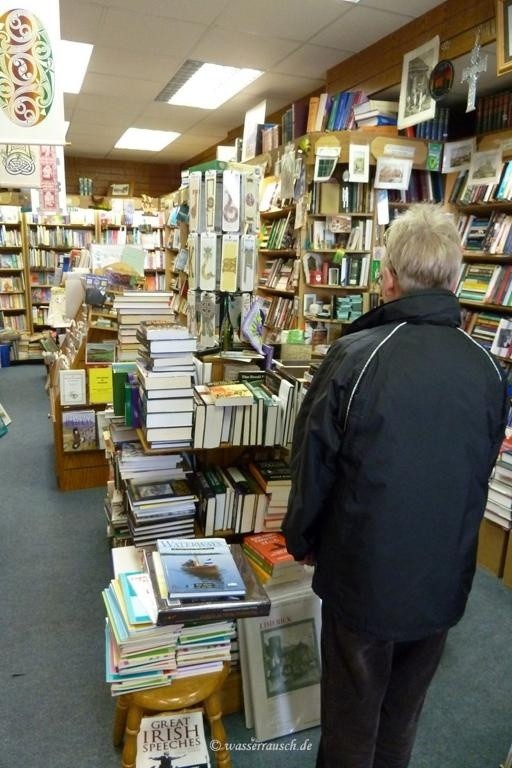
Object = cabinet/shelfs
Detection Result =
[4,128,510,716]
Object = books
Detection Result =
[83,176,88,195]
[100,540,324,768]
[165,85,512,540]
[78,177,84,196]
[0,196,165,363]
[87,177,93,196]
[43,363,165,540]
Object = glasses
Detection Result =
[381,224,397,280]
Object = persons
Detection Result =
[274,196,511,767]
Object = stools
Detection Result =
[110,662,234,767]
